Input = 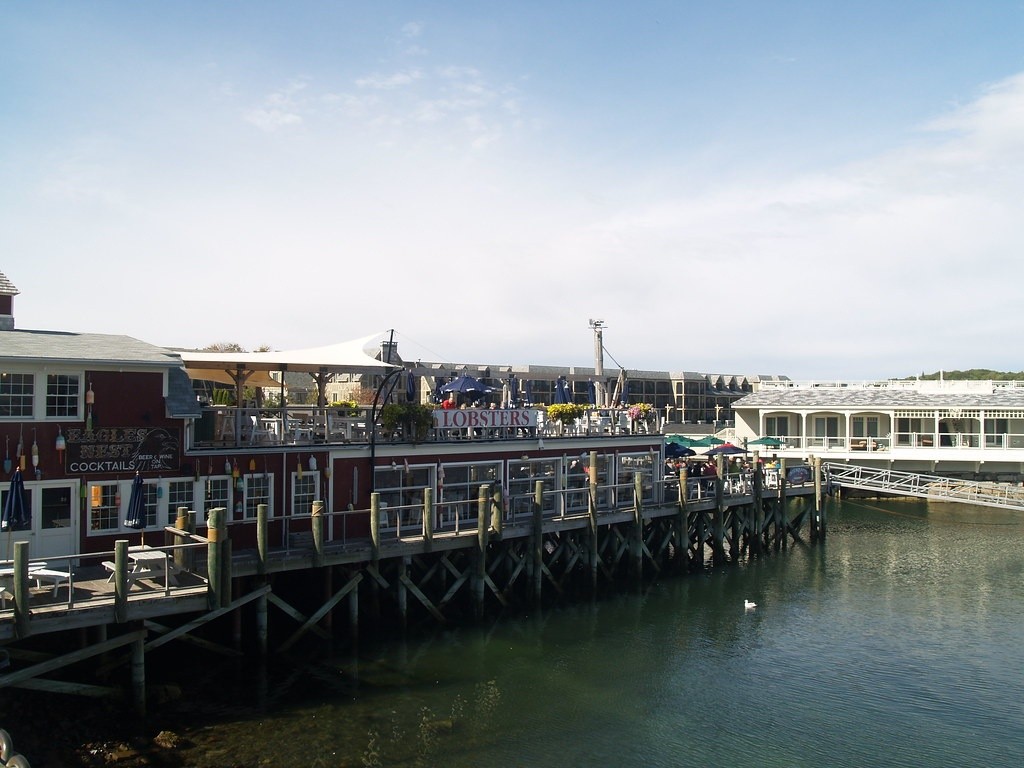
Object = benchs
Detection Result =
[28,568,75,598]
[0,587,6,609]
[102,560,179,577]
[851,435,933,450]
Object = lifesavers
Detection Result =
[788,468,807,484]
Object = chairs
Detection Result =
[377,464,780,530]
[219,409,632,447]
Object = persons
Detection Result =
[666,458,779,481]
[600,401,627,432]
[442,398,499,434]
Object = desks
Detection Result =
[331,416,371,444]
[1,559,47,608]
[107,545,179,594]
[261,417,301,447]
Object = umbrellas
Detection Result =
[407,371,415,404]
[124,473,147,548]
[434,372,628,404]
[664,432,785,457]
[2,470,29,561]
